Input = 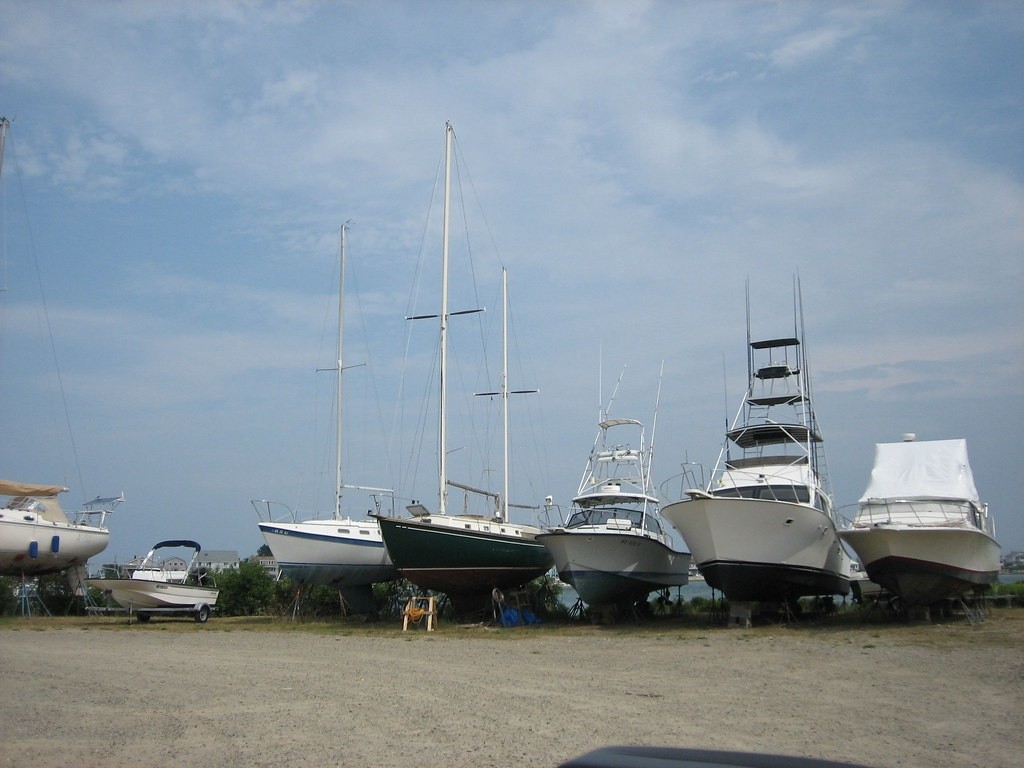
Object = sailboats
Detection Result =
[257,223,395,587]
[370,123,559,595]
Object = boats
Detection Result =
[0,484,122,579]
[850,560,894,601]
[661,276,856,608]
[538,362,695,608]
[835,436,1001,610]
[88,540,218,624]
